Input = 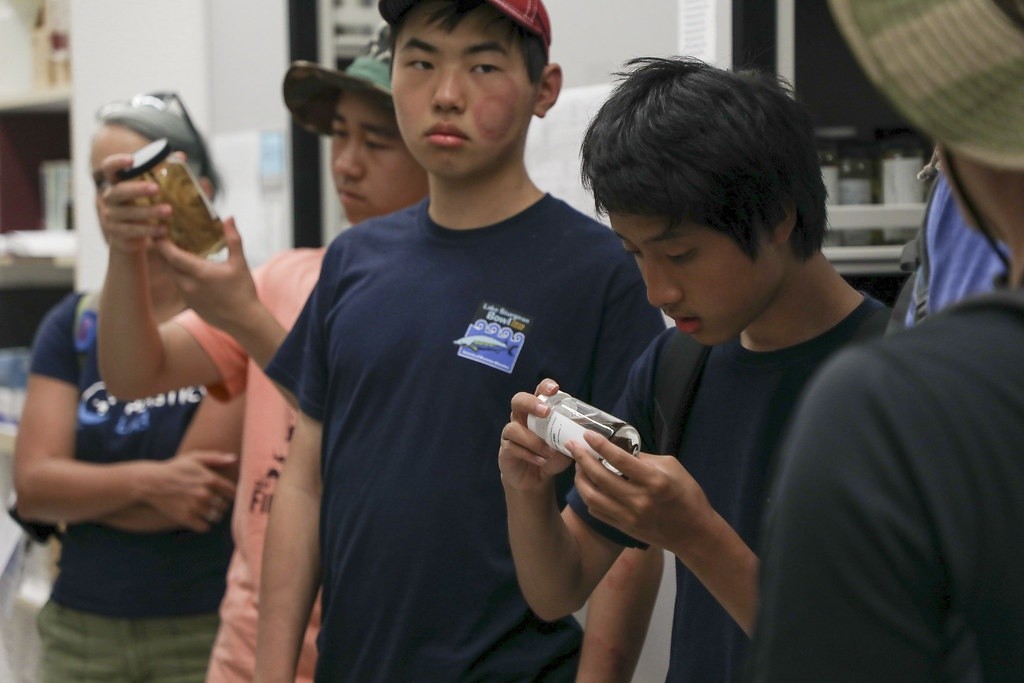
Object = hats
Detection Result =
[832,0,1024,172]
[377,0,552,66]
[284,23,396,135]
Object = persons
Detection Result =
[498,54,892,682]
[99,24,430,683]
[740,0,1024,683]
[257,0,666,683]
[883,141,1009,333]
[13,90,246,683]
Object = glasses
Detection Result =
[135,91,210,177]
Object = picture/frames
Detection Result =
[287,0,773,254]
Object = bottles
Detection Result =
[528,390,640,476]
[819,123,928,247]
[116,138,228,257]
[40,149,73,230]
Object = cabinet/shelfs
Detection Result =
[2,0,209,288]
